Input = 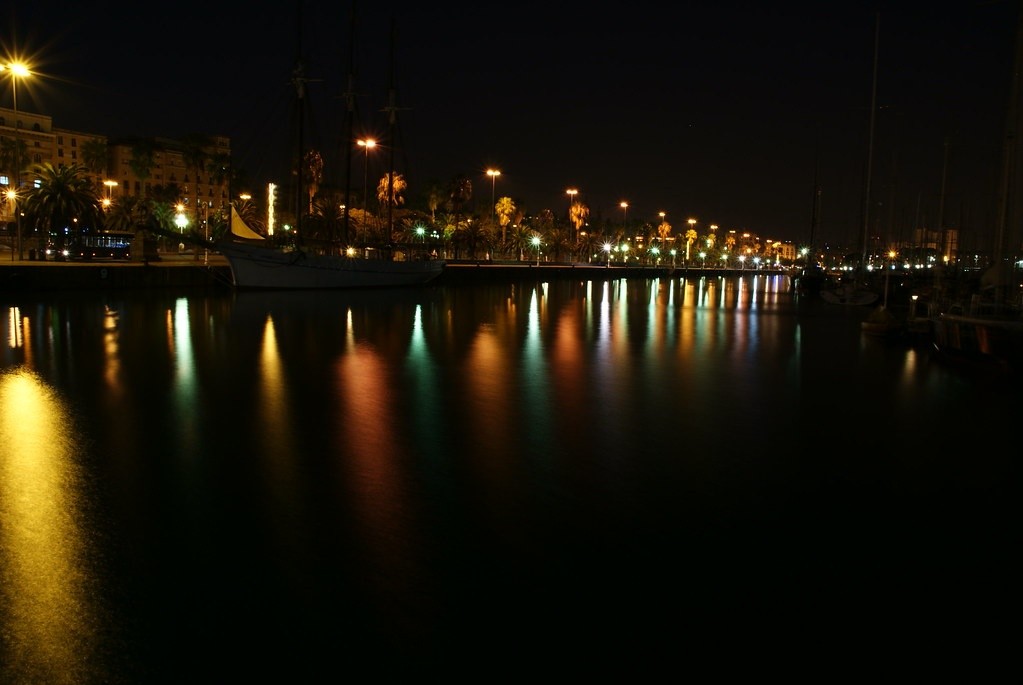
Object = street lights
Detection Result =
[4,61,36,260]
[566,188,578,242]
[621,202,628,242]
[484,169,501,234]
[356,137,377,246]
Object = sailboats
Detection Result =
[128,56,449,293]
[791,7,1022,364]
